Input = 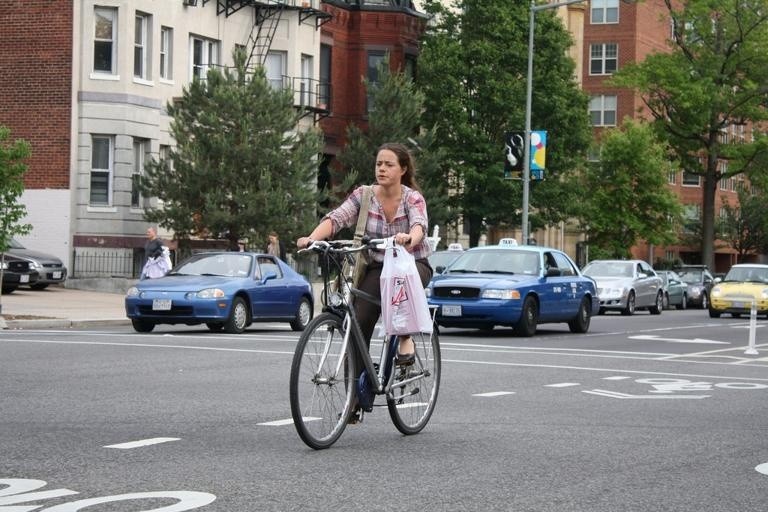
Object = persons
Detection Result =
[296,143,433,425]
[145,226,165,280]
[266,231,286,263]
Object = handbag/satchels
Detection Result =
[334,252,367,289]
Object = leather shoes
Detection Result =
[395,339,415,365]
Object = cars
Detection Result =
[125,252,314,334]
[0,233,67,294]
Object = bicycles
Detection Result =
[289,237,441,450]
